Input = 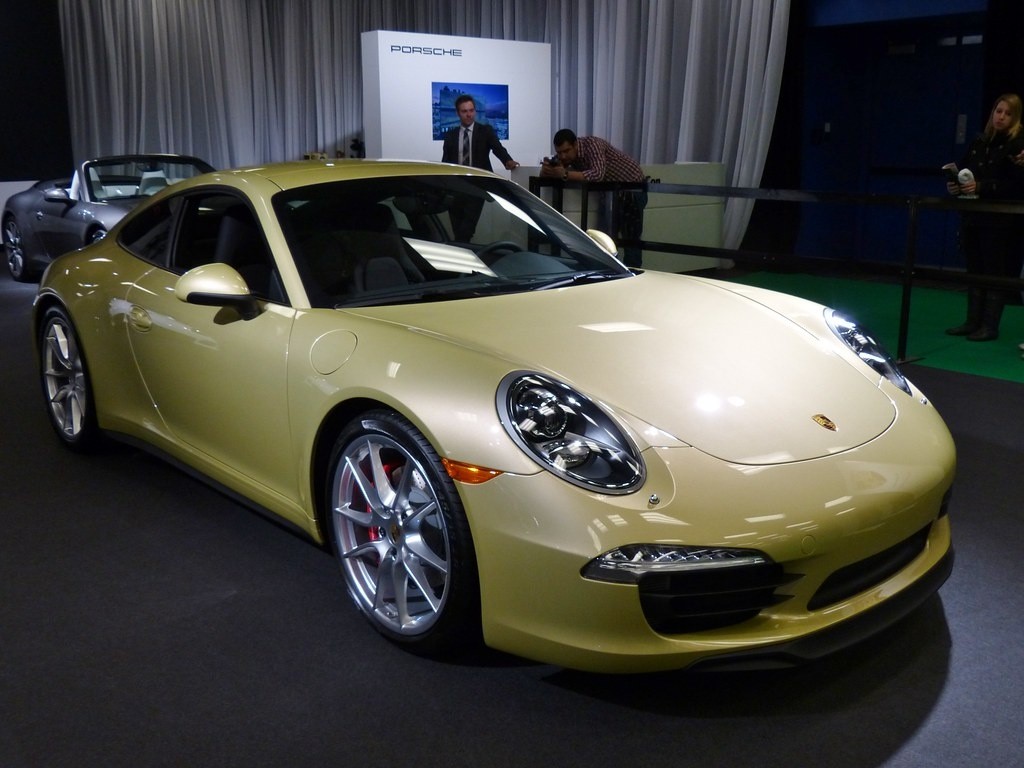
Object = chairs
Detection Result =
[301,204,427,297]
[138,169,170,197]
[69,161,105,200]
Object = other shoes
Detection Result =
[969,326,999,341]
[945,320,976,335]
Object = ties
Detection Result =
[462,128,470,166]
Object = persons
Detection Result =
[441,95,520,243]
[539,129,648,268]
[944,92,1024,342]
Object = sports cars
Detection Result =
[28,157,960,671]
[1,153,218,285]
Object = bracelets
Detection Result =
[562,171,569,182]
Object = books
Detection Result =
[943,162,965,195]
